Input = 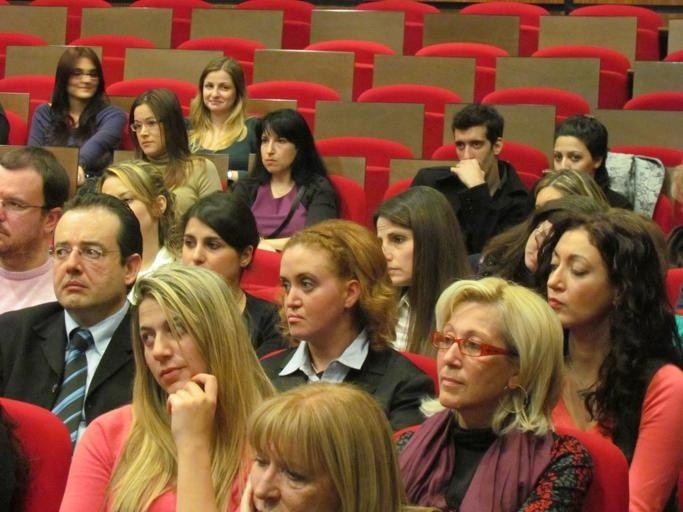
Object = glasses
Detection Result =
[433,331,508,357]
[3,199,44,213]
[131,120,160,132]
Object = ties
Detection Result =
[50,329,93,450]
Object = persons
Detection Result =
[0,46,682,512]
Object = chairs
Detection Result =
[1,0,683,512]
